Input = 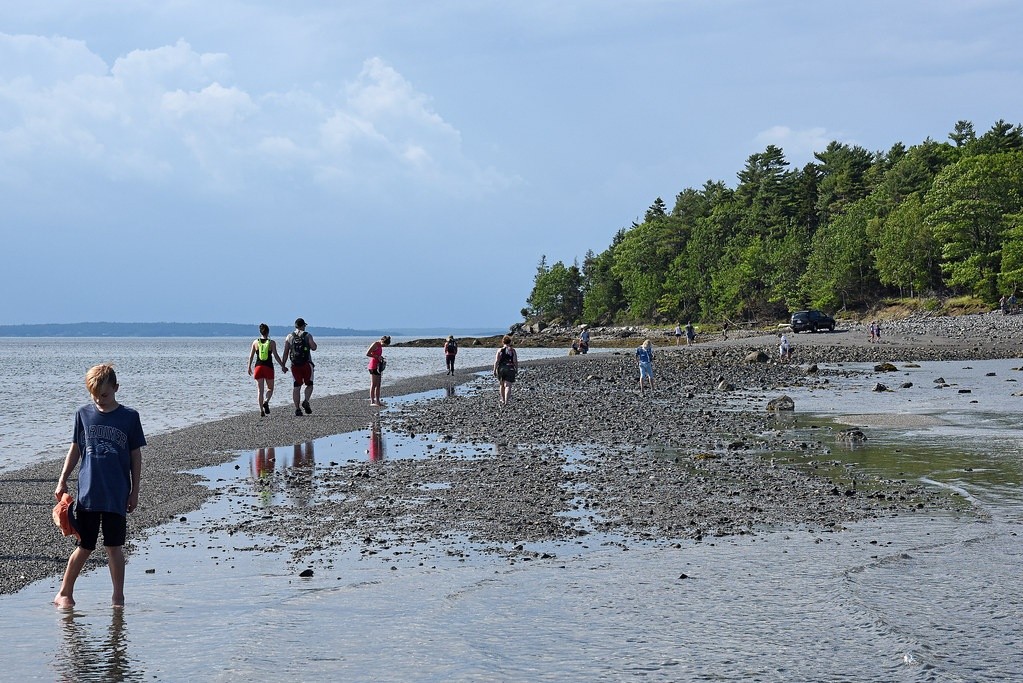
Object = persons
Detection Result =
[494,335,518,405]
[674,323,683,347]
[281,318,317,416]
[685,321,695,347]
[777,333,791,364]
[248,324,288,416]
[1006,294,1017,315]
[721,319,728,341]
[635,340,656,398]
[868,322,876,343]
[53,362,148,605]
[366,335,391,406]
[875,325,883,343]
[572,327,590,354]
[999,295,1008,316]
[445,335,457,376]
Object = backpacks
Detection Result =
[447,341,455,353]
[256,338,271,360]
[290,331,309,365]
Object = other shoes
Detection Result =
[446,371,450,375]
[295,408,302,416]
[263,401,270,414]
[260,411,265,416]
[451,373,454,375]
[302,399,311,414]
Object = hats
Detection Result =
[52,493,81,543]
[446,335,454,341]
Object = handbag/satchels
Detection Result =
[377,358,385,372]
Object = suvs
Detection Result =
[789,309,836,333]
